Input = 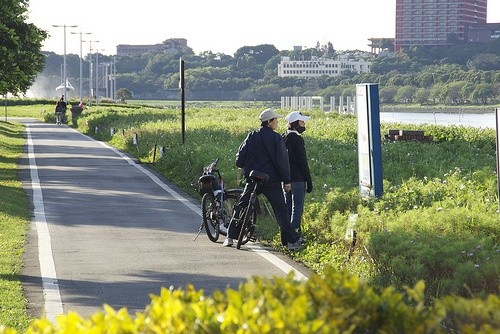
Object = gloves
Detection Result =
[306,180,312,193]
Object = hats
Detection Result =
[285,111,310,123]
[259,109,282,122]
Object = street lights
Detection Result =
[72,32,92,101]
[82,41,100,98]
[52,25,78,101]
[91,49,105,105]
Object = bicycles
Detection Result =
[224,167,269,249]
[193,158,239,242]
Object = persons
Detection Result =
[222,108,304,250]
[55,97,66,125]
[280,111,313,246]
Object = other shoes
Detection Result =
[287,237,306,249]
[223,238,233,247]
